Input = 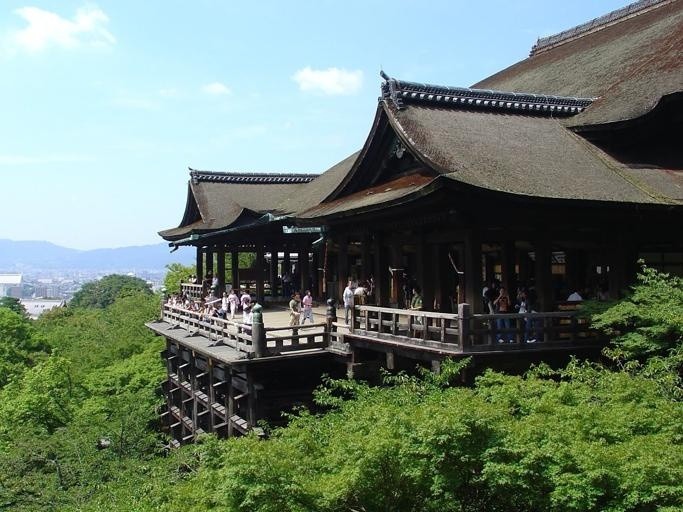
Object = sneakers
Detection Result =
[497,337,538,343]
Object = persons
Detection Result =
[281,271,290,298]
[337,270,611,346]
[300,289,317,330]
[293,291,302,325]
[162,270,255,336]
[288,294,301,331]
